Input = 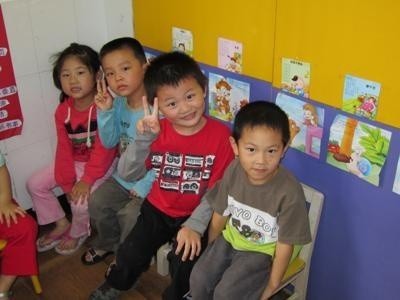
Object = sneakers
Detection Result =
[89,277,139,300]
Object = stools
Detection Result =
[0,238,43,295]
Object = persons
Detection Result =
[186,97,314,300]
[0,148,41,299]
[87,48,239,300]
[24,40,121,258]
[81,35,166,280]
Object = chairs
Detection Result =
[150,156,326,300]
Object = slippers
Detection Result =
[81,246,114,265]
[105,259,119,278]
[56,232,90,256]
[37,223,70,252]
